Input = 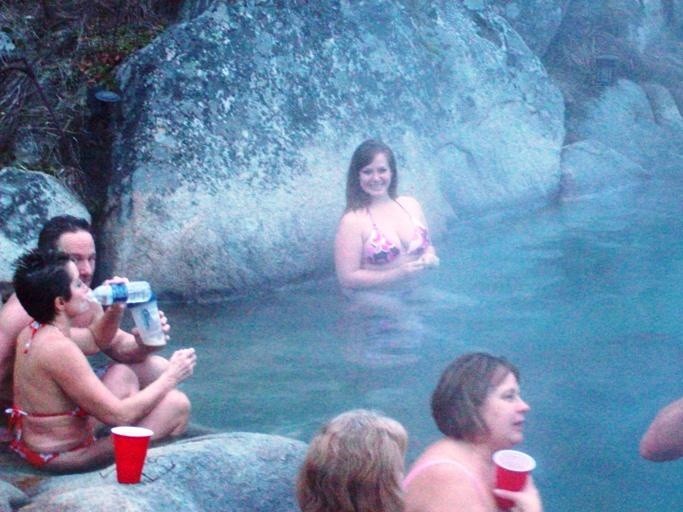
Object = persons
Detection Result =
[334,139,496,353]
[295,408,405,512]
[638,396,683,463]
[395,353,542,512]
[0,215,170,447]
[5,250,198,475]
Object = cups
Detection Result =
[110,426,153,484]
[127,289,167,347]
[492,449,536,509]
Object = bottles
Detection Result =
[89,281,152,305]
